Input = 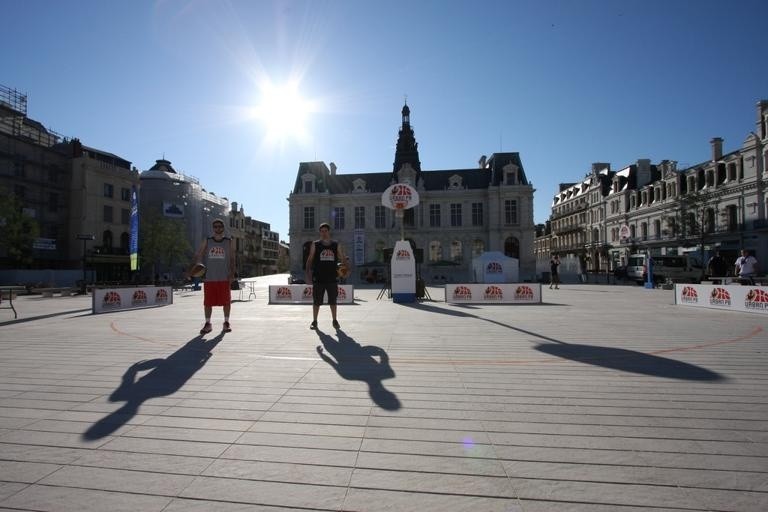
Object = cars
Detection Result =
[615,266,626,279]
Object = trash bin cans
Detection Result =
[542,272,550,284]
[580,272,588,284]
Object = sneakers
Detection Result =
[332,319,340,328]
[310,320,317,329]
[223,321,231,331]
[200,321,212,333]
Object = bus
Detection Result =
[627,254,703,283]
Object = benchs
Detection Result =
[0,285,156,300]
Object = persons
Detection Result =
[155,272,159,286]
[188,219,236,333]
[734,248,758,285]
[708,251,728,284]
[549,259,561,289]
[306,222,349,329]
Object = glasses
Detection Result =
[213,225,224,229]
